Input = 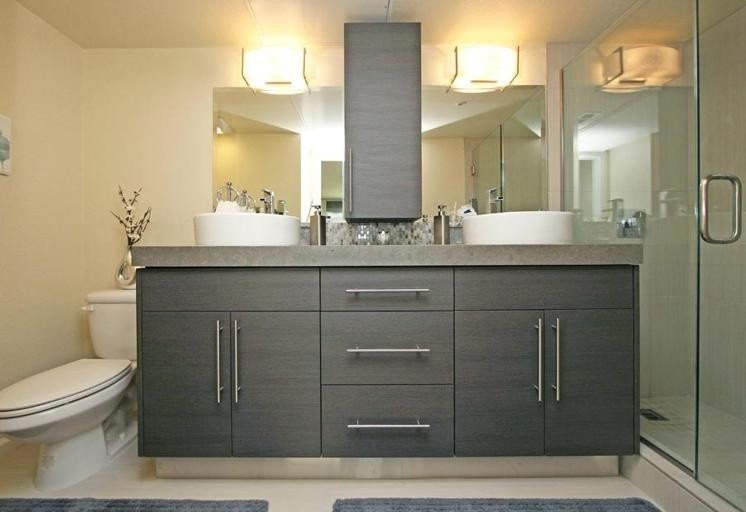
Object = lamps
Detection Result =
[602,44,683,89]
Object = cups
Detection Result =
[353,224,392,244]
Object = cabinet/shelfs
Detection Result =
[343,20,424,223]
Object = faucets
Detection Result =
[467,198,479,215]
[599,198,625,222]
[485,187,504,214]
[277,200,288,214]
[258,187,275,213]
[656,189,681,218]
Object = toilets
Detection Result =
[0,288,140,494]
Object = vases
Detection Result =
[114,245,136,290]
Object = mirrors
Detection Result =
[210,84,551,225]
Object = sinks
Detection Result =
[461,210,573,245]
[638,211,746,244]
[193,212,301,247]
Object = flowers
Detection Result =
[109,184,153,246]
